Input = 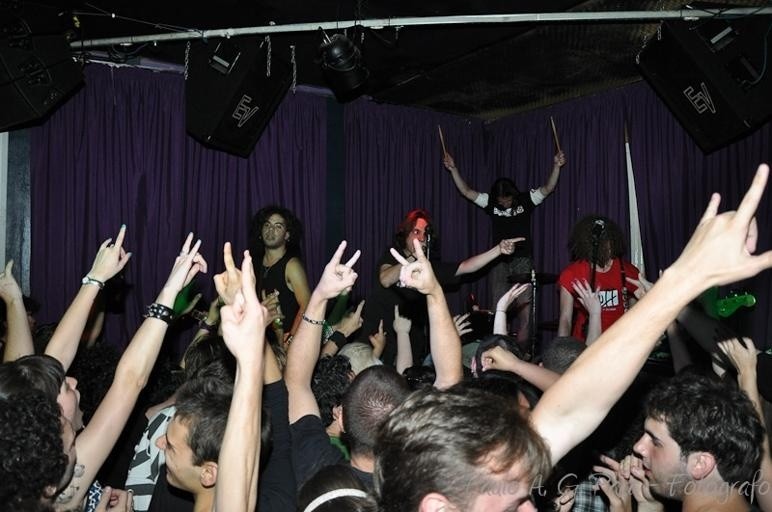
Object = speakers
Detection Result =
[637,19,772,156]
[0,0,86,133]
[184,37,297,161]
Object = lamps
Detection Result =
[318,26,377,104]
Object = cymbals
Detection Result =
[538,320,559,331]
[506,273,559,285]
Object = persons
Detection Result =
[3,149,772,512]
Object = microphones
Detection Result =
[594,219,604,235]
[425,225,433,234]
